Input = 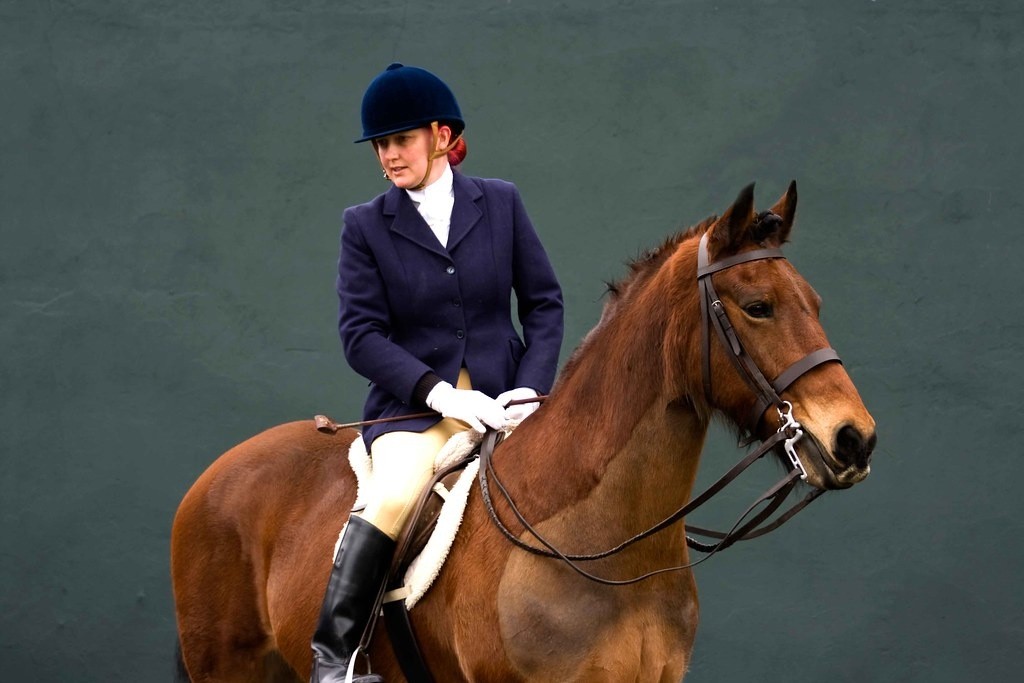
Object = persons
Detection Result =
[311,64,565,683]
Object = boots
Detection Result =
[310,513,397,682]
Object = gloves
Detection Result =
[495,387,540,429]
[425,381,511,434]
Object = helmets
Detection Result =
[354,62,465,144]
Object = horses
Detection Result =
[169,177,878,683]
